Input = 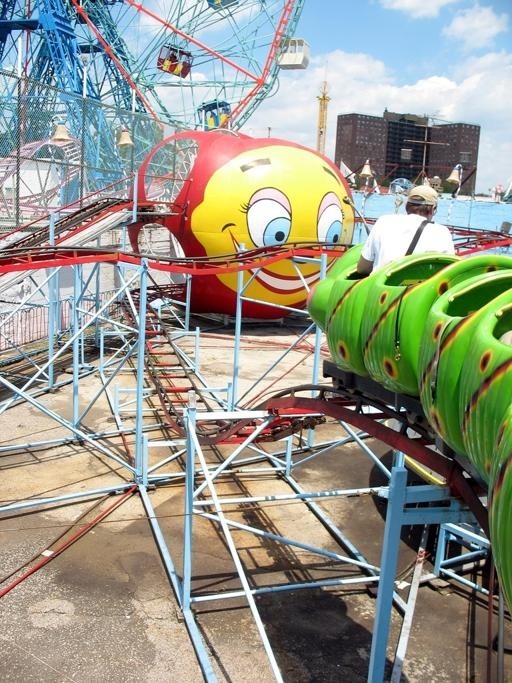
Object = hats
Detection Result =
[407,186,438,206]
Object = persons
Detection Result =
[357,185,458,277]
